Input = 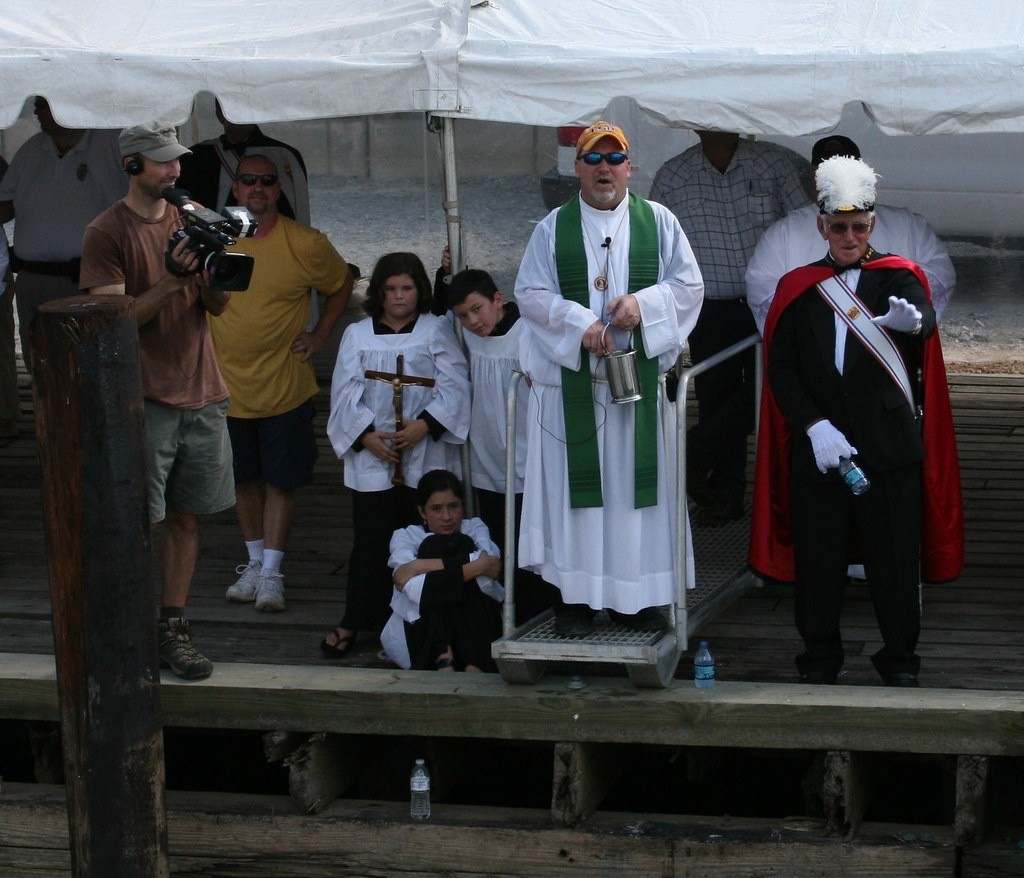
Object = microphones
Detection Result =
[605,237,611,249]
[189,225,224,252]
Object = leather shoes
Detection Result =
[798,672,828,684]
[885,672,918,688]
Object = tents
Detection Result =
[0,0,1024,519]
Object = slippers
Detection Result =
[320,628,355,655]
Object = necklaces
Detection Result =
[579,204,629,291]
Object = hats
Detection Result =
[118,120,192,163]
[576,120,631,154]
[815,156,879,215]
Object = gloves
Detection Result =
[870,296,922,333]
[807,418,859,474]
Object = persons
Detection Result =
[80,119,239,680]
[431,246,552,628]
[379,469,504,675]
[0,96,313,437]
[514,121,705,637]
[647,129,957,586]
[209,154,353,612]
[749,151,967,687]
[321,252,473,659]
[374,375,422,422]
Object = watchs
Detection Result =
[908,321,922,335]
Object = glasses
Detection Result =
[235,173,277,187]
[826,219,871,235]
[577,151,628,165]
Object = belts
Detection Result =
[17,260,76,276]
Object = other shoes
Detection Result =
[696,506,734,523]
[607,608,666,630]
[555,608,592,636]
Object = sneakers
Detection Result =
[227,561,261,601]
[157,616,214,679]
[255,569,286,611]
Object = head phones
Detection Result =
[121,159,142,174]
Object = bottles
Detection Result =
[838,455,870,495]
[409,760,430,820]
[695,642,715,689]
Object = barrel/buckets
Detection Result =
[601,321,644,404]
